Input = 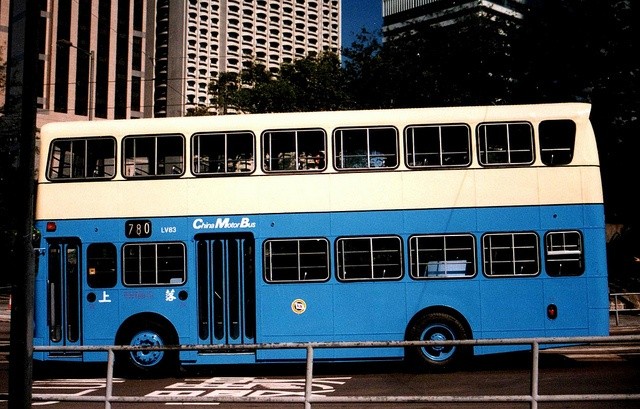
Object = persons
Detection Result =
[313,152,323,170]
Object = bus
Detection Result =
[32,102,611,380]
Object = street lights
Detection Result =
[56,40,95,121]
